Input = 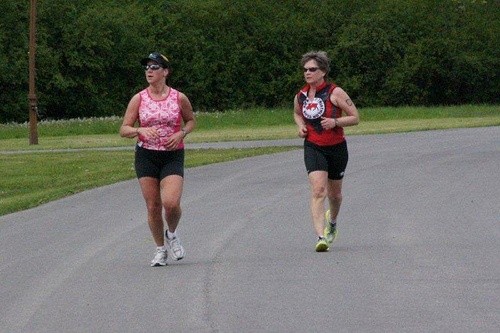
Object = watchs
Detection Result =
[182,128,188,136]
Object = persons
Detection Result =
[293,52,360,253]
[118,52,195,268]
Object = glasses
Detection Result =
[302,67,320,72]
[144,65,161,70]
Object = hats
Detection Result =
[141,52,171,69]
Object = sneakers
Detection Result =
[150,248,168,267]
[165,229,186,261]
[315,235,329,252]
[323,209,338,244]
